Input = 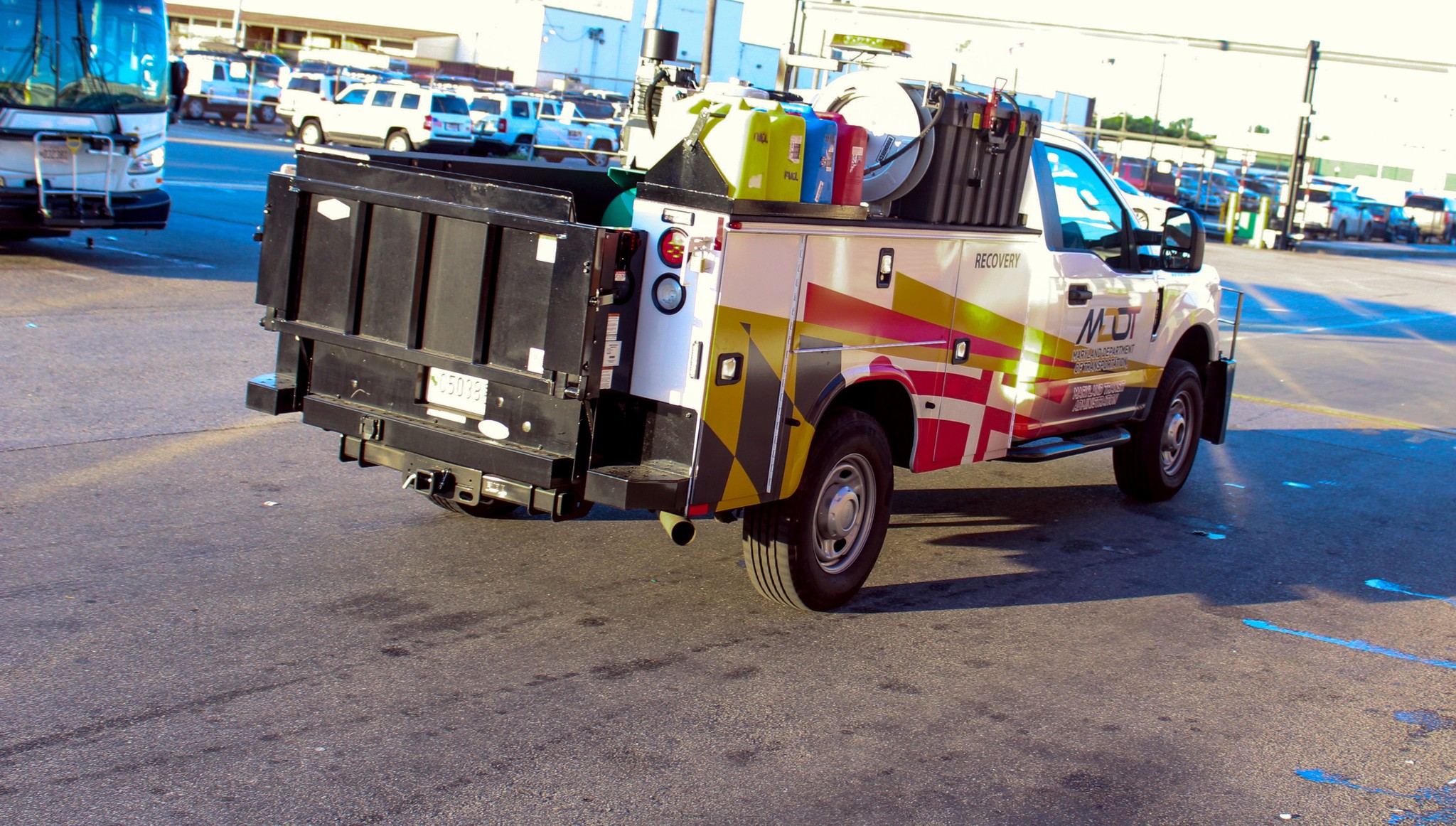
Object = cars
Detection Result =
[1157,162,1288,217]
[1361,201,1422,244]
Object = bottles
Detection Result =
[672,82,867,206]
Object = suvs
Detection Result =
[297,77,476,154]
[466,86,619,166]
[1278,184,1373,241]
[172,47,336,123]
[1400,194,1456,244]
[1091,150,1181,205]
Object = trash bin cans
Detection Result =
[1270,220,1282,241]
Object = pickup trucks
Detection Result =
[245,57,1243,615]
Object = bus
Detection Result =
[0,0,192,245]
